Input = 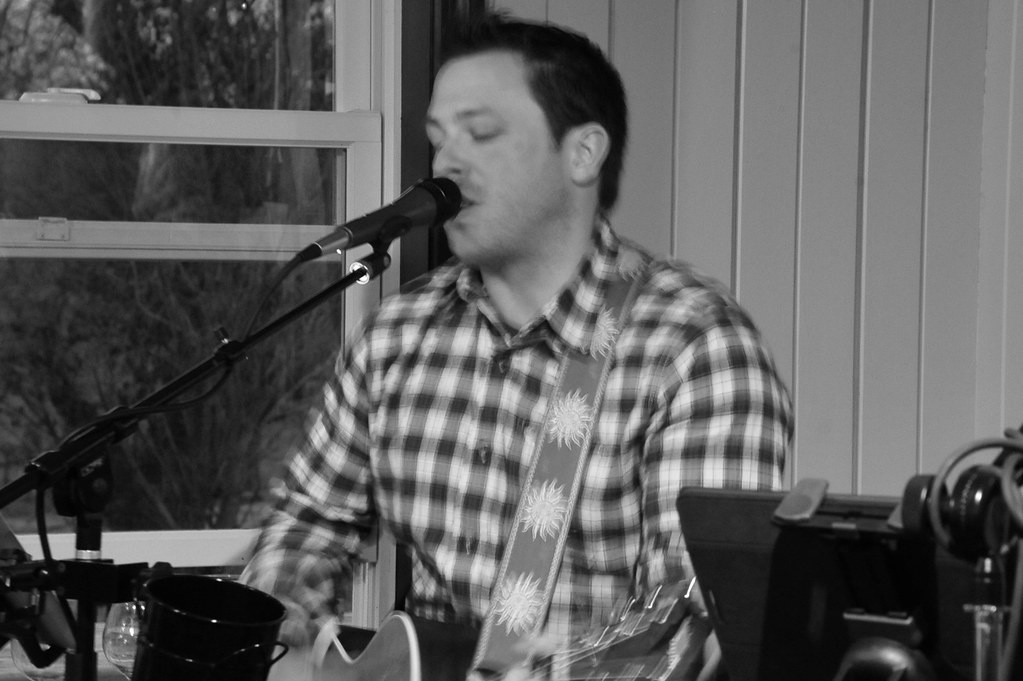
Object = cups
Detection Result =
[102,601,146,681]
[129,574,288,681]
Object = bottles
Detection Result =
[74,518,103,562]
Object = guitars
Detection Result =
[308,572,698,681]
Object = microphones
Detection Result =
[297,175,461,264]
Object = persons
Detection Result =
[237,18,795,681]
